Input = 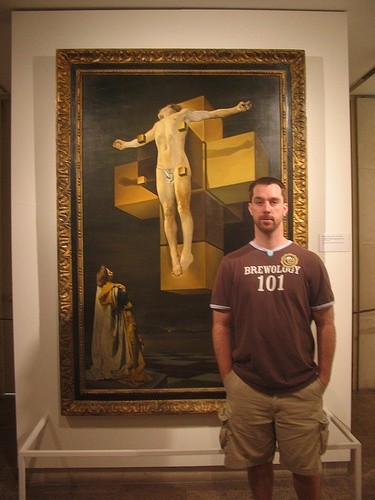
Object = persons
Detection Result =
[111,100,253,277]
[208,176,337,500]
[83,265,152,389]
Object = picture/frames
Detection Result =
[53,47,310,417]
